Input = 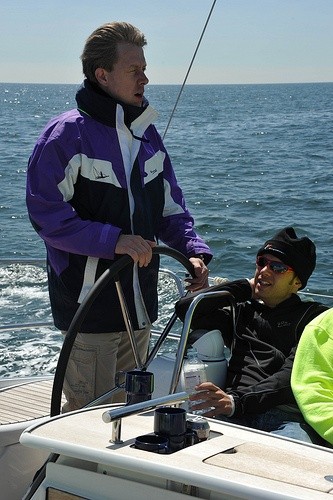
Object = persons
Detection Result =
[25,21,214,414]
[174,227,333,449]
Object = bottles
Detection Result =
[183,349,211,415]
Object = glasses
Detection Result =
[255,255,295,274]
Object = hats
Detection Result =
[257,226,316,290]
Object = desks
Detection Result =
[19,403,333,500]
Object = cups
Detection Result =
[116,370,154,407]
[154,408,210,451]
[135,436,169,454]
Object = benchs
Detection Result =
[1,377,68,448]
